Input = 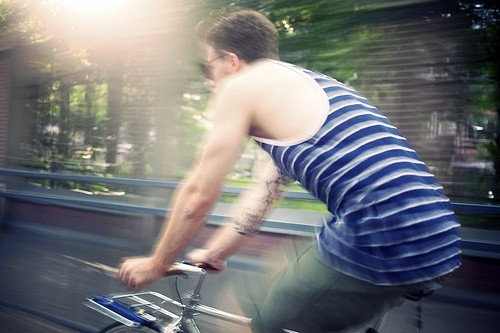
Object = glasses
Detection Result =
[199,54,231,81]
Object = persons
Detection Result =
[119,10,464,333]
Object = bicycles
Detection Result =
[84,260,360,333]
